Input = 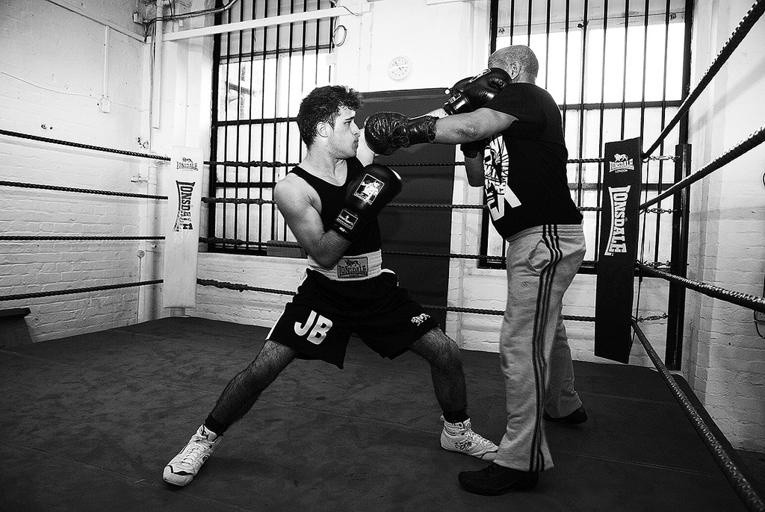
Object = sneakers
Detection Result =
[162,424,224,486]
[440,417,500,462]
[458,463,540,497]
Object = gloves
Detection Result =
[332,164,403,241]
[442,67,512,160]
[364,111,437,157]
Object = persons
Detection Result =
[364,44,587,496]
[161,84,500,489]
